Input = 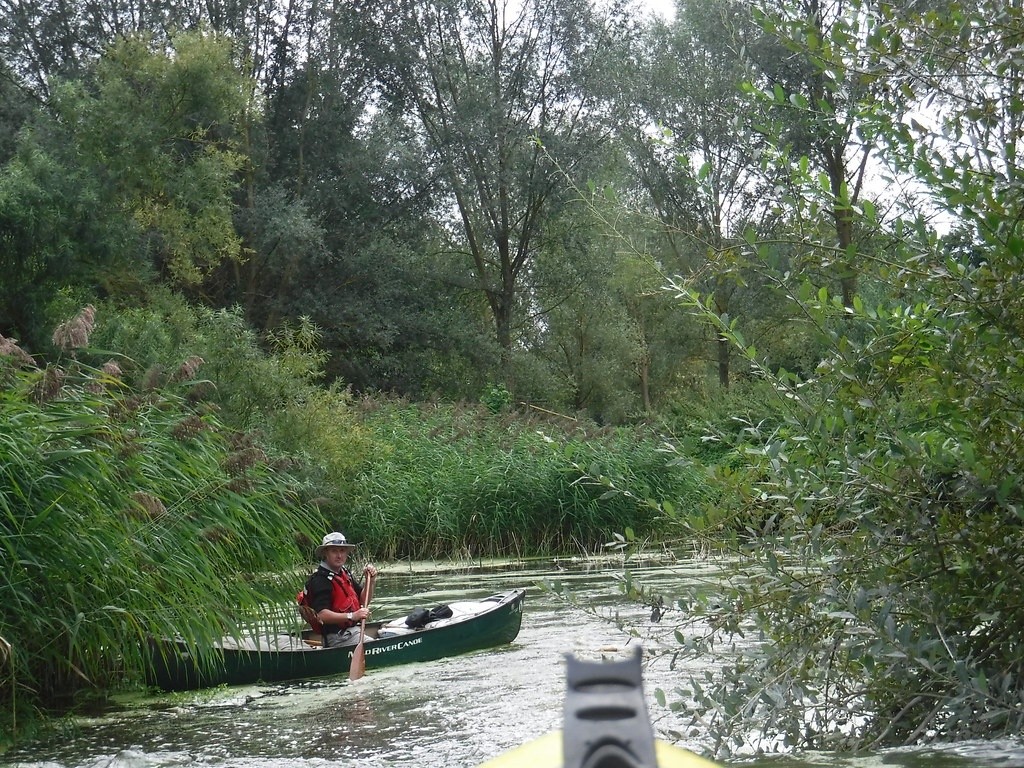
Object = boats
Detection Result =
[142,588,525,687]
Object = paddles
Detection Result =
[349,569,371,682]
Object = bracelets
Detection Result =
[347,610,353,626]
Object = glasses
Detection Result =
[325,540,348,545]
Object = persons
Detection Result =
[304,532,378,648]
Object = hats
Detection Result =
[315,532,355,559]
